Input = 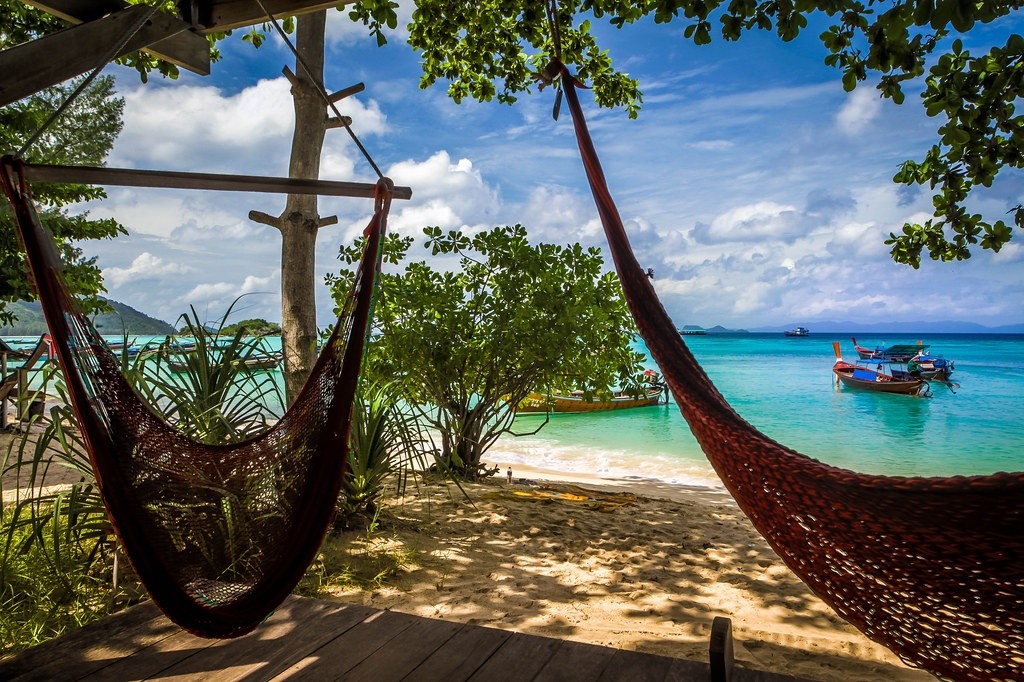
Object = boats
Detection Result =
[851,335,933,365]
[168,351,284,373]
[499,369,671,415]
[832,340,930,397]
[784,327,810,337]
[907,349,955,382]
